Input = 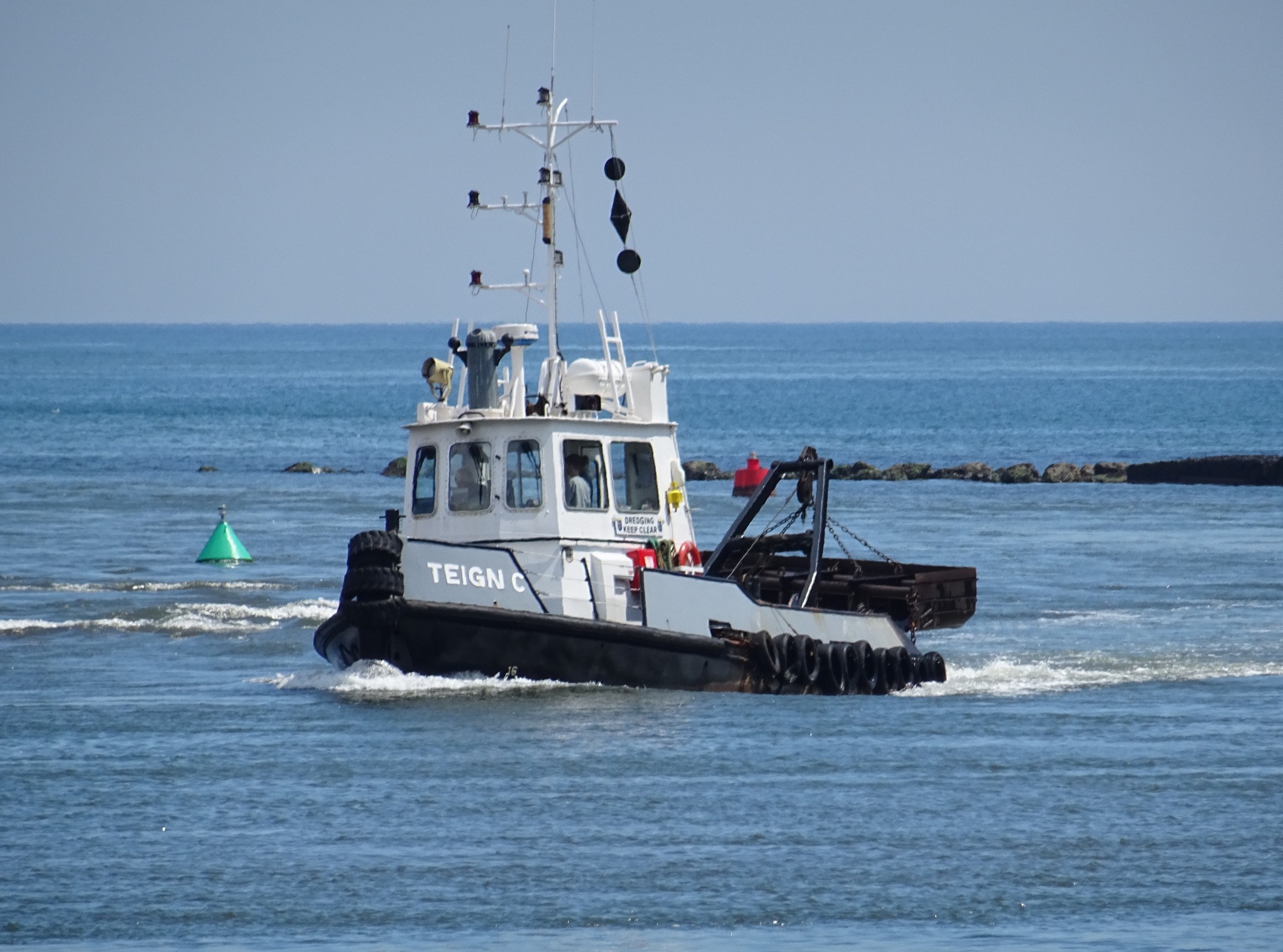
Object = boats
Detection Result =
[311,78,978,696]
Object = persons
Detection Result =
[563,454,591,508]
[579,455,593,508]
[454,467,485,498]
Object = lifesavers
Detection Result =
[677,541,703,575]
[751,630,947,696]
